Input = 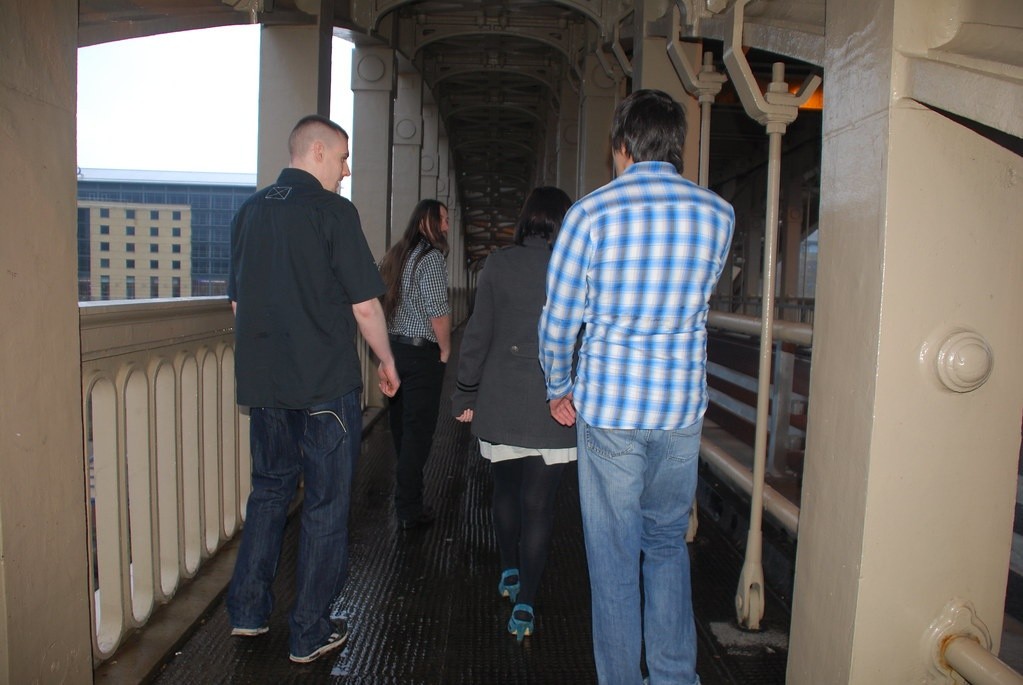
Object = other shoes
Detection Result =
[400,514,434,529]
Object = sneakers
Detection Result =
[231,626,270,636]
[289,619,348,663]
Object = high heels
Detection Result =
[507,602,534,642]
[498,569,521,603]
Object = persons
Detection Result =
[537,89,736,685]
[226,115,401,662]
[453,186,586,641]
[377,199,452,531]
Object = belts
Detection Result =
[388,332,429,347]
